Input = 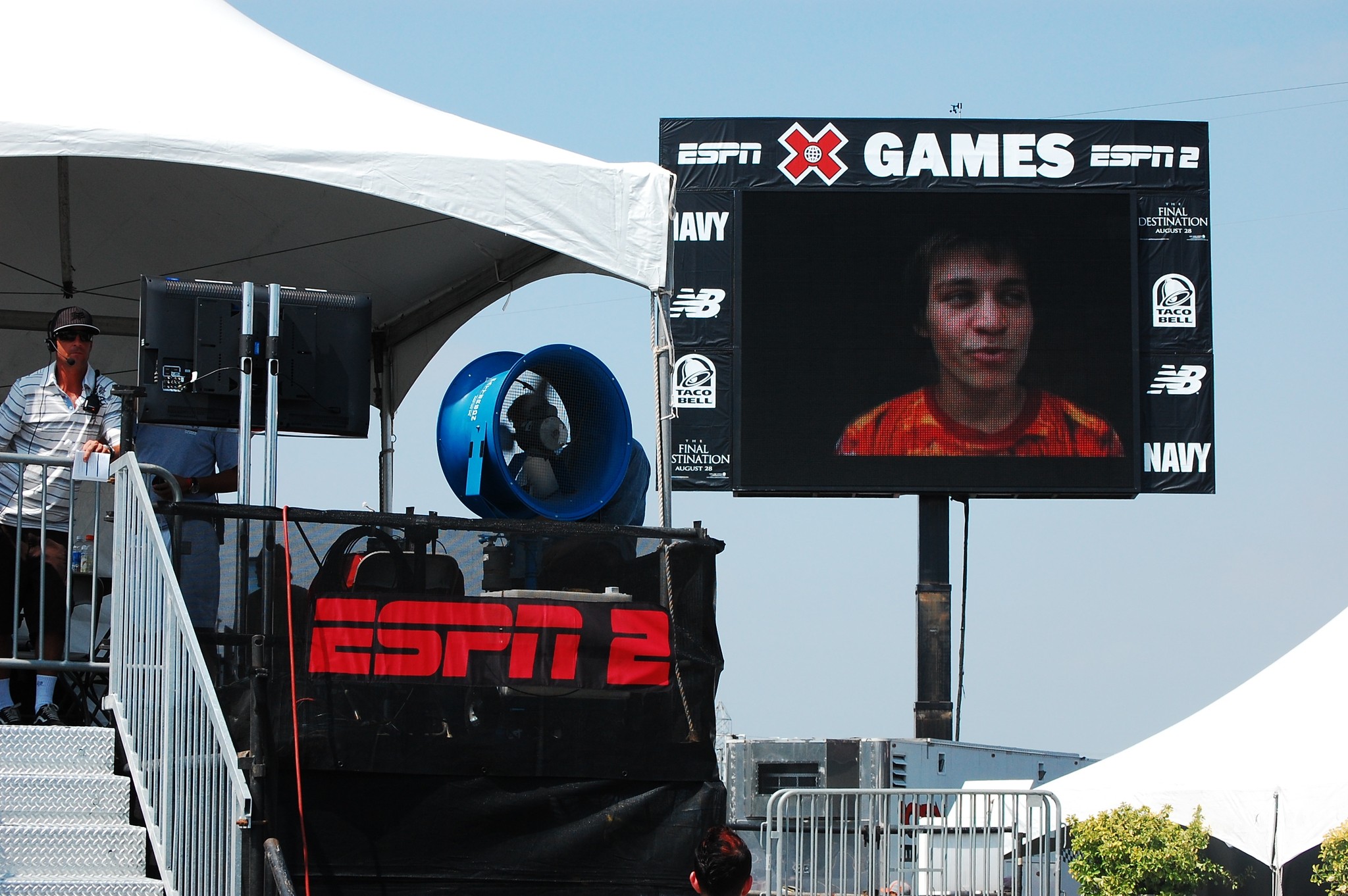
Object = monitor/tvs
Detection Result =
[139,274,373,438]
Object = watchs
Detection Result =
[189,474,200,497]
[103,443,115,459]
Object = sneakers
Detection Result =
[32,703,62,725]
[0,703,22,725]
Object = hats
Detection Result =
[51,306,100,339]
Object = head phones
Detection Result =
[44,306,92,352]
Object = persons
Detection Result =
[689,822,752,896]
[133,420,239,660]
[242,542,312,676]
[0,306,122,725]
[881,880,911,896]
[829,218,1128,464]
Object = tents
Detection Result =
[0,0,677,552]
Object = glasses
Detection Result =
[54,331,93,342]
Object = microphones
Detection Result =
[47,339,76,366]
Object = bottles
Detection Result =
[80,535,94,573]
[72,535,84,572]
